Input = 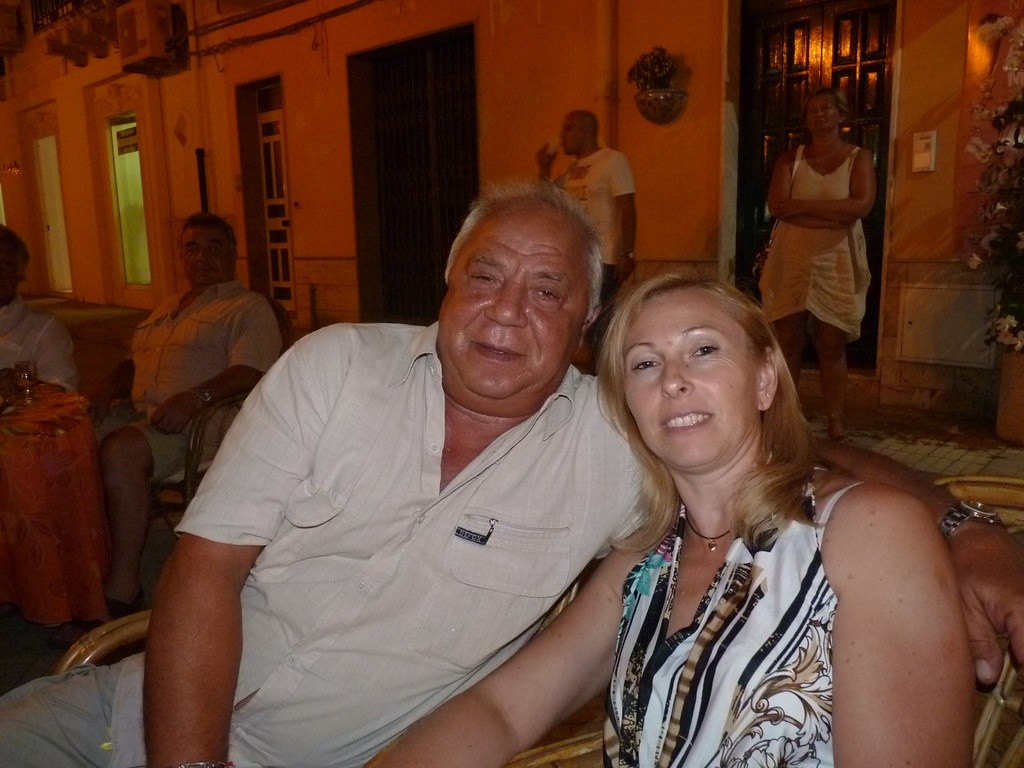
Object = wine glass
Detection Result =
[14,361,40,409]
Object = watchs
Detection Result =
[941,502,1005,539]
[196,384,211,407]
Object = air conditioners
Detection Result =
[116,0,177,73]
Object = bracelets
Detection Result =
[179,761,236,768]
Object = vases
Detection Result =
[633,89,686,125]
[993,353,1024,445]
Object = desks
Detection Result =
[0,372,106,627]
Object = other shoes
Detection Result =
[46,583,145,655]
[828,416,855,446]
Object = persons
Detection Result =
[758,86,876,440]
[1,224,94,400]
[535,110,636,371]
[49,212,285,648]
[2,182,1023,767]
[338,270,976,768]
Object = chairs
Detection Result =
[151,294,295,508]
[515,474,1023,768]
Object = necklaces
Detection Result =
[684,506,730,552]
[806,143,843,169]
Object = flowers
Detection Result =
[951,93,1024,358]
[627,47,676,91]
